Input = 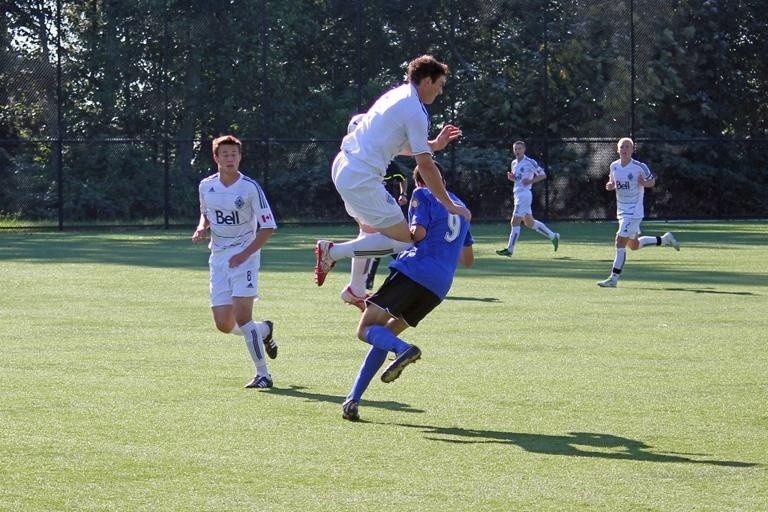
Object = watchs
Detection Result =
[401,192,407,196]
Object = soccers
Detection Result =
[347,113,365,133]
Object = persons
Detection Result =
[367,163,409,289]
[314,54,473,311]
[495,140,561,257]
[342,161,473,418]
[596,137,682,288]
[189,133,279,389]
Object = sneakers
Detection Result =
[552,233,560,251]
[262,320,278,359]
[664,231,680,251]
[245,374,273,387]
[315,240,422,419]
[597,279,618,287]
[496,248,512,257]
